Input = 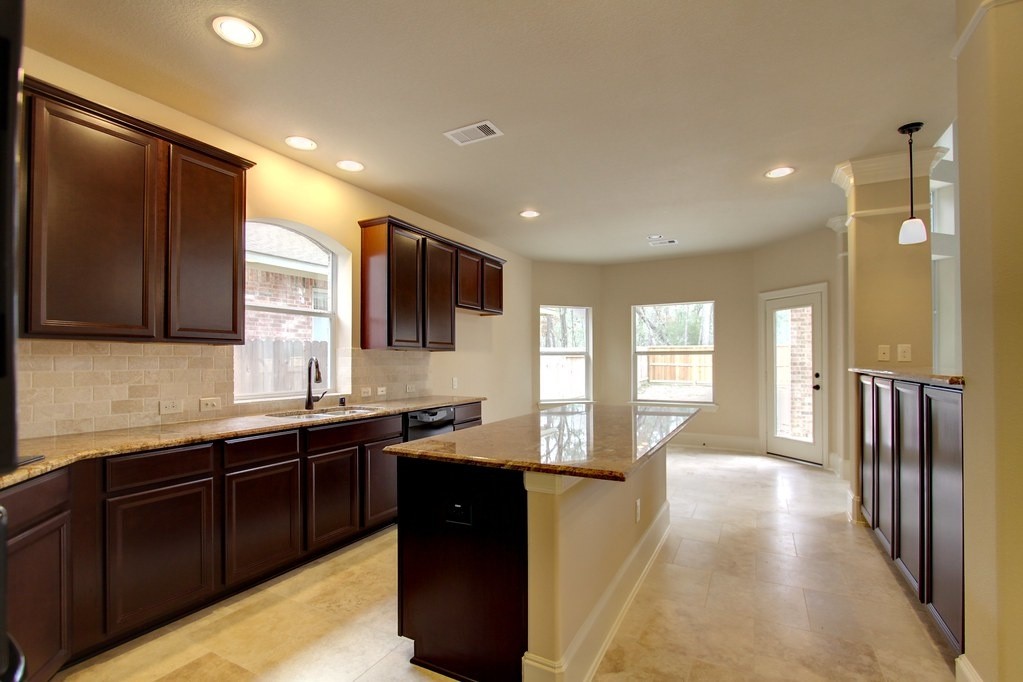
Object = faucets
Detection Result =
[305,356,327,411]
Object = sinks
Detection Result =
[264,413,332,419]
[320,408,374,416]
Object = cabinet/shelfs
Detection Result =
[0,400,482,682]
[397,456,528,682]
[19,73,257,344]
[858,373,965,657]
[358,214,507,352]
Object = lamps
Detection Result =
[897,121,927,245]
[212,16,263,49]
